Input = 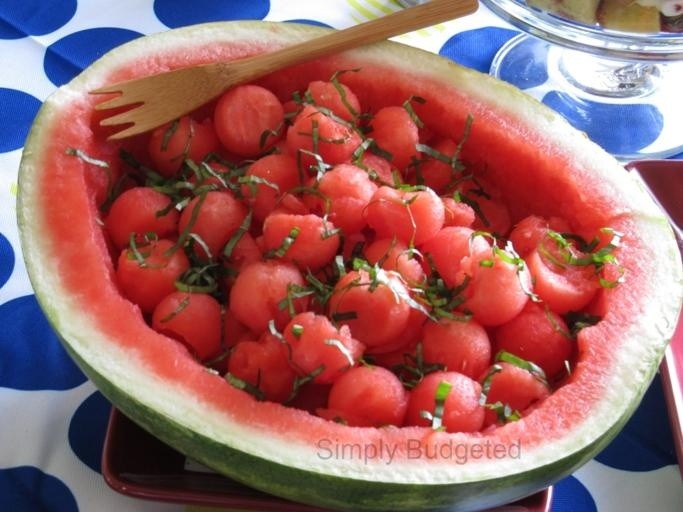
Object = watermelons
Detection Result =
[15,20,683,512]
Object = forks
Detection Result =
[88,0,482,145]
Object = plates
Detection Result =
[98,401,560,511]
[619,157,681,485]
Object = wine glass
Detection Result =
[480,0,683,163]
